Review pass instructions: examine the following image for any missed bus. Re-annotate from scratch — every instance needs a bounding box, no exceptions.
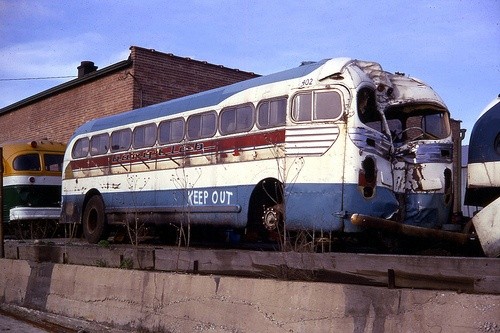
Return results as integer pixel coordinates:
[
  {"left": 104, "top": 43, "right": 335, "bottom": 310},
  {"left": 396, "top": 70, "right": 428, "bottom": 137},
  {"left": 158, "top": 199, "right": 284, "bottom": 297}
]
[
  {"left": 1, "top": 138, "right": 67, "bottom": 235},
  {"left": 59, "top": 56, "right": 457, "bottom": 243}
]
